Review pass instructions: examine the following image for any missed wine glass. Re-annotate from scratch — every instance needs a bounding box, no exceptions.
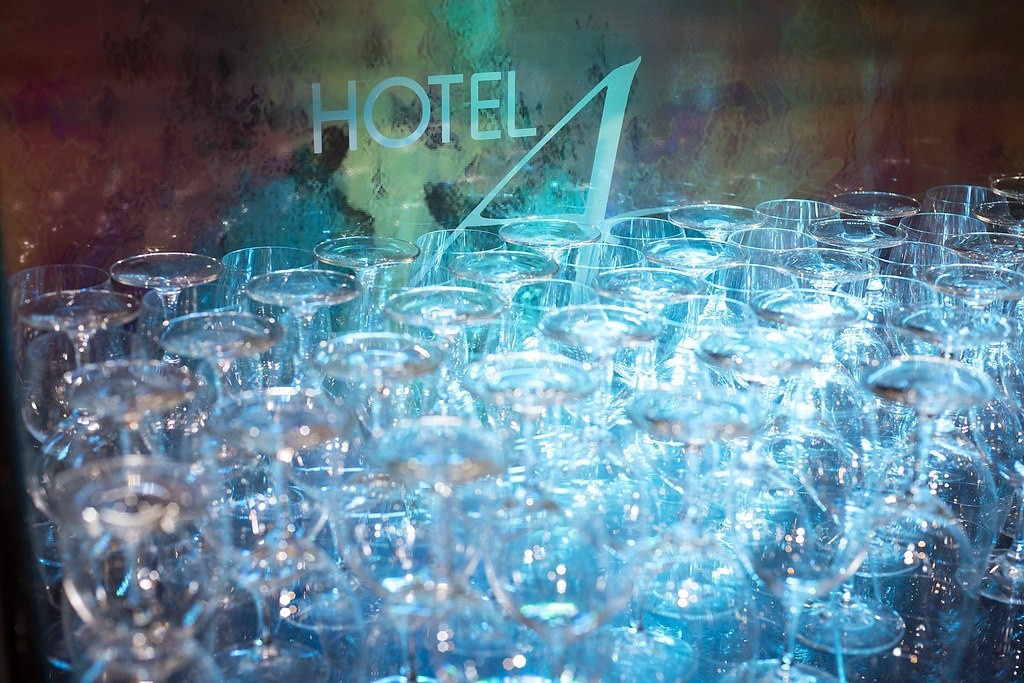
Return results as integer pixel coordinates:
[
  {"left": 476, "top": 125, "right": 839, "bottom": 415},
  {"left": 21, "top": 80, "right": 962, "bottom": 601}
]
[{"left": 7, "top": 173, "right": 1024, "bottom": 683}]
